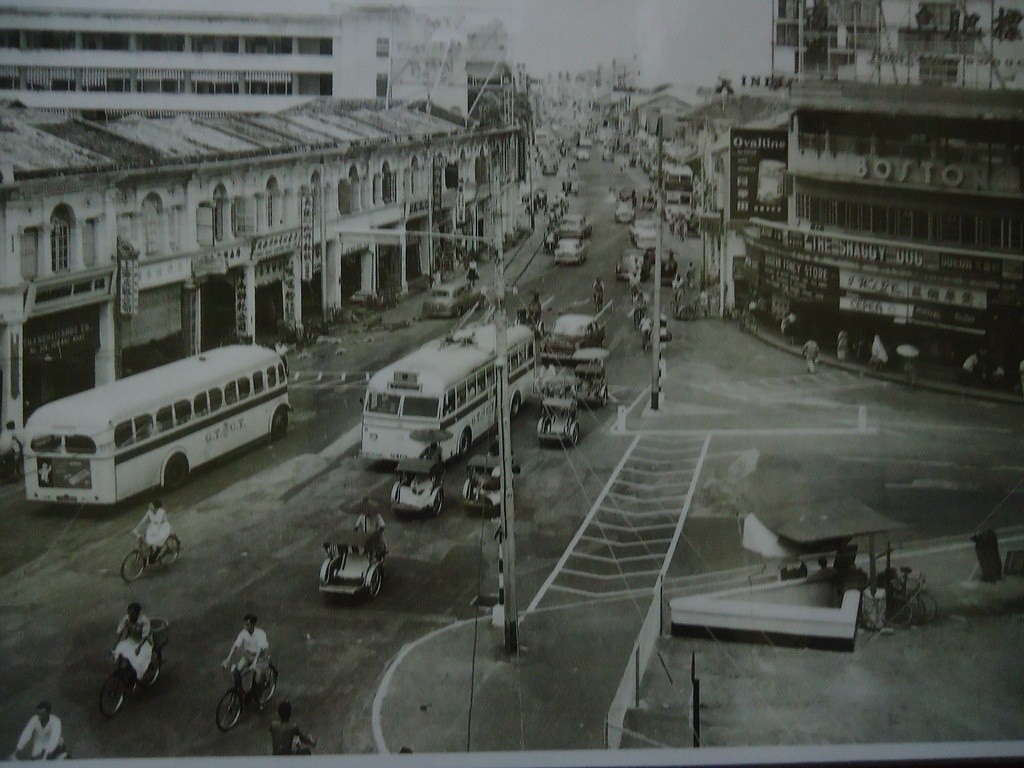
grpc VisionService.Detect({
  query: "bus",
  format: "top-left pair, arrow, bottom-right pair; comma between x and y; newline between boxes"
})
18,340 -> 295,507
356,222 -> 561,473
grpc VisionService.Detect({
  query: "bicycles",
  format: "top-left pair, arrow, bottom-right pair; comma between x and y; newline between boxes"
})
6,746 -> 72,763
593,287 -> 603,312
640,329 -> 651,353
632,303 -> 647,331
291,730 -> 317,755
96,615 -> 171,718
120,530 -> 183,584
879,566 -> 939,627
215,652 -> 280,732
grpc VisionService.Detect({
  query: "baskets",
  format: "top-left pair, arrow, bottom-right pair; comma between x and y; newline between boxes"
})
149,618 -> 169,647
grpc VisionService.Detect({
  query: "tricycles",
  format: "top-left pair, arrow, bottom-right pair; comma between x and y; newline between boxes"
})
390,426 -> 455,516
462,436 -> 521,510
570,347 -> 611,407
513,305 -> 547,341
316,496 -> 391,606
535,374 -> 582,447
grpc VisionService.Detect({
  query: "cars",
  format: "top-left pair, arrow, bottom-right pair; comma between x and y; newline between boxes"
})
541,313 -> 608,368
420,278 -> 482,320
614,248 -> 653,283
535,136 -> 660,268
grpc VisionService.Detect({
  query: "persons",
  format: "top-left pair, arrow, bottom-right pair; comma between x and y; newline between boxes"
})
533,138 -> 578,253
631,151 -> 713,240
133,498 -> 170,564
0,420 -> 23,474
9,701 -> 68,760
399,746 -> 413,754
639,312 -> 652,335
354,513 -> 387,560
628,251 -> 709,317
572,348 -> 611,360
269,700 -> 316,755
559,383 -> 576,400
529,294 -> 542,321
488,435 -> 500,454
538,357 -> 556,398
113,603 -> 154,693
466,258 -> 479,287
222,614 -> 270,710
780,312 -> 1024,395
419,441 -> 442,461
476,468 -> 489,486
592,277 -> 604,305
432,268 -> 442,290
589,354 -> 604,368
808,556 -> 840,583
274,341 -> 288,373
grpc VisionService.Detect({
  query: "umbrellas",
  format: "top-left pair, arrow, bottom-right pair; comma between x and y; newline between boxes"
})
543,374 -> 580,397
339,496 -> 389,533
409,429 -> 454,447
896,344 -> 919,368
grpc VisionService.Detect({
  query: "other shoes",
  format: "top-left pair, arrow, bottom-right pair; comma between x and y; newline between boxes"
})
150,555 -> 156,564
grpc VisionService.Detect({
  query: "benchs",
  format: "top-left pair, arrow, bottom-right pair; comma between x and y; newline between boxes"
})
333,553 -> 370,583
547,416 -> 570,434
